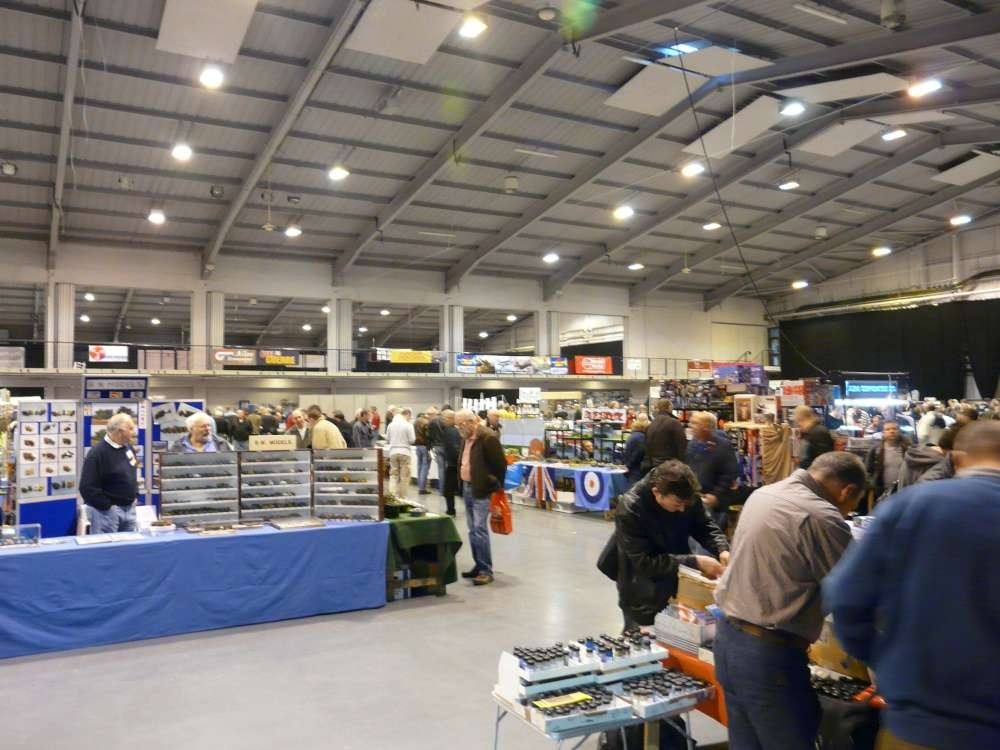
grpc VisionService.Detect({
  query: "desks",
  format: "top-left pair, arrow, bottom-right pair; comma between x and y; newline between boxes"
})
0,507 -> 463,657
632,621 -> 885,750
505,460 -> 628,518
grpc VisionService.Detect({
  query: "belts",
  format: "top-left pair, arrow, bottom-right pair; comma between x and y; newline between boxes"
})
727,616 -> 809,650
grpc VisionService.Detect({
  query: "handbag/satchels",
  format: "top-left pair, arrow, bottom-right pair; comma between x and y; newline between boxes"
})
490,489 -> 511,535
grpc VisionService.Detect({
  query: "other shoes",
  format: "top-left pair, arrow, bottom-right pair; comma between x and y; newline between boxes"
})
473,575 -> 491,585
462,569 -> 477,578
419,490 -> 431,494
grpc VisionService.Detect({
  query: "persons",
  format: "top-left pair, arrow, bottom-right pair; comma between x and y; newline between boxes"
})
616,461 -> 730,630
573,400 -> 652,425
385,405 -> 461,516
171,413 -> 230,515
625,399 -> 737,555
79,414 -> 139,534
455,409 -> 507,584
213,404 -> 380,504
479,410 -> 502,438
706,452 -> 869,750
824,419 -> 1000,750
793,400 -> 1000,515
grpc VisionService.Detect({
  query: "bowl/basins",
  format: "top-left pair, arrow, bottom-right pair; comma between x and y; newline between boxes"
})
754,414 -> 774,424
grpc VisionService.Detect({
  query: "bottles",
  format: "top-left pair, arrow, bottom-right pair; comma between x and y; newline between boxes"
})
6,512 -> 15,525
462,392 -> 540,419
512,603 -> 716,717
741,402 -> 748,421
